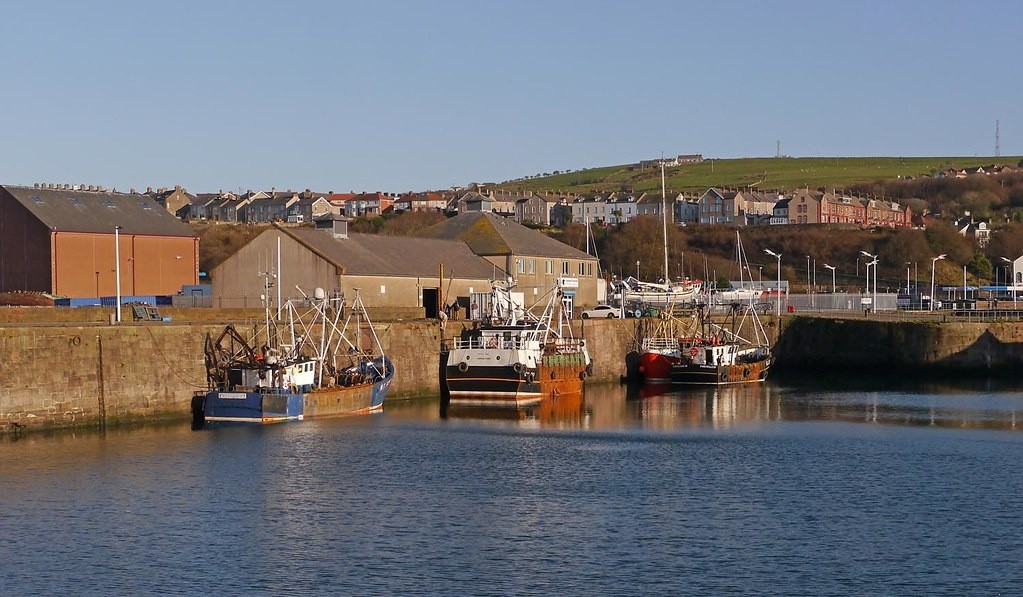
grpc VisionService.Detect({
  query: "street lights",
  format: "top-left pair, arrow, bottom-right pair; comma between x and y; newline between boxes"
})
765,243 -> 948,315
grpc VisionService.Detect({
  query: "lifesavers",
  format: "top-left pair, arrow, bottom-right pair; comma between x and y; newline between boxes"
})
712,336 -> 719,347
690,347 -> 698,356
489,338 -> 497,347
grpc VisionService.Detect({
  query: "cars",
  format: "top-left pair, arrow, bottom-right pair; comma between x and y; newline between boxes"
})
897,293 -> 1023,308
581,304 -> 622,320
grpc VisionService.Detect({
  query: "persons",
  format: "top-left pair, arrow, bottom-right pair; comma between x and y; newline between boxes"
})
471,300 -> 478,320
443,301 -> 451,320
994,297 -> 998,308
450,299 -> 460,321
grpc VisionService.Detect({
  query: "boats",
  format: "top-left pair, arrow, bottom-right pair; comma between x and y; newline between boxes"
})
632,293 -> 775,389
195,246 -> 396,429
445,274 -> 595,408
